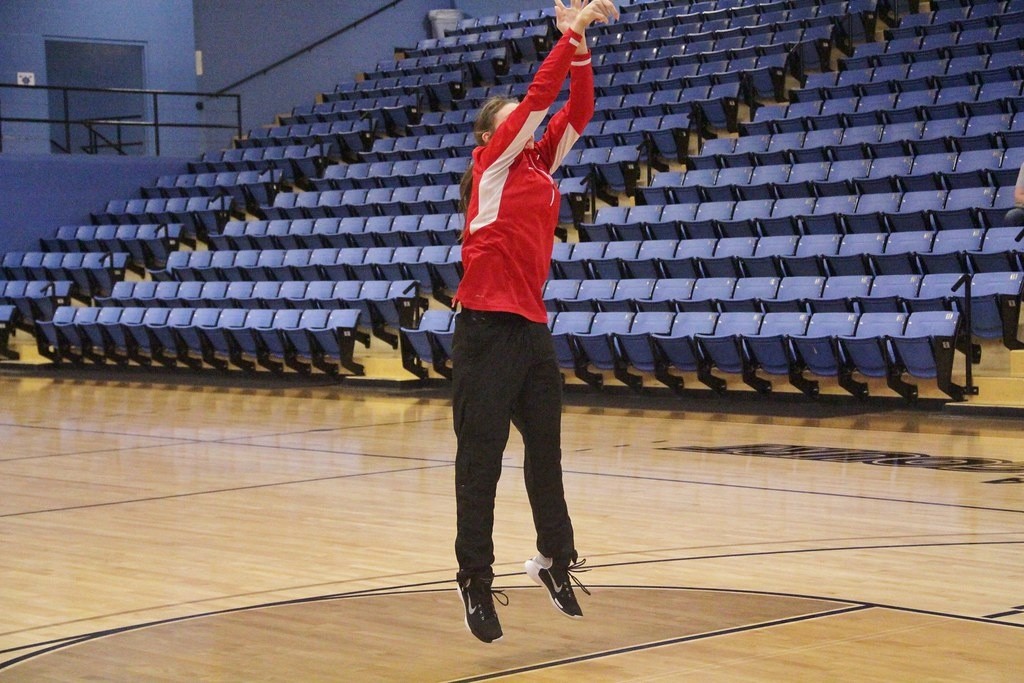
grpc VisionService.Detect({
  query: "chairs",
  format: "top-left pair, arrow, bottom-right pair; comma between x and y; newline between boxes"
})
0,0 -> 1024,400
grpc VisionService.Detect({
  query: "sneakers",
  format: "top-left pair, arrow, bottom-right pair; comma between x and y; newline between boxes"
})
525,552 -> 591,620
456,578 -> 509,644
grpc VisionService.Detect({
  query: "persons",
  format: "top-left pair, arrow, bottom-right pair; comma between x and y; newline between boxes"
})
451,0 -> 620,643
1004,163 -> 1024,226
